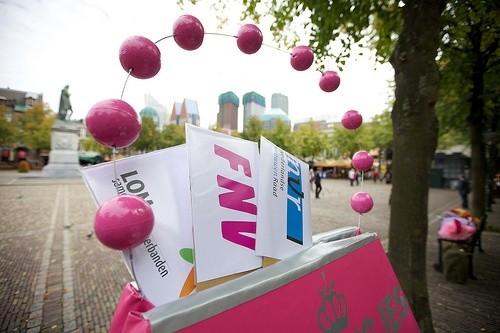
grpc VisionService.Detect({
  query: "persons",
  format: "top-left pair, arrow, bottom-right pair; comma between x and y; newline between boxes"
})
58,84 -> 73,120
308,166 -> 379,197
457,174 -> 471,208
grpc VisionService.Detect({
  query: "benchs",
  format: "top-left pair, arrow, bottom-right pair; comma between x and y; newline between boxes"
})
433,211 -> 487,281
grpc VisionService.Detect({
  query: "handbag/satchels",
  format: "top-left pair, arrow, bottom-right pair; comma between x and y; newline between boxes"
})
442,244 -> 470,282
